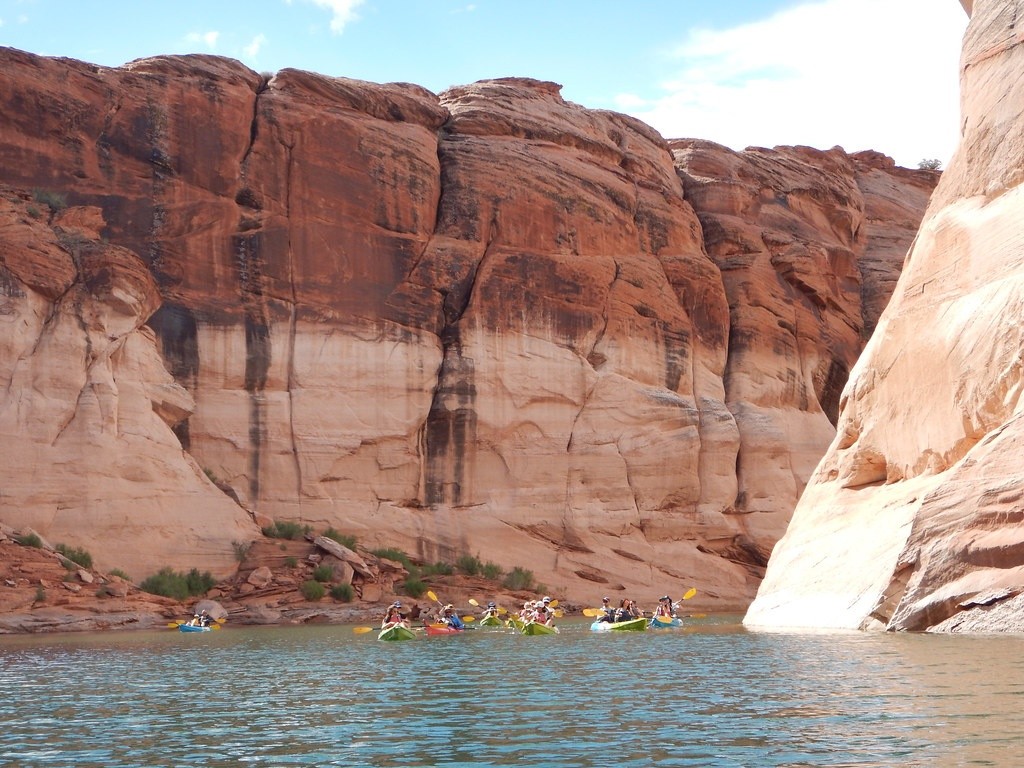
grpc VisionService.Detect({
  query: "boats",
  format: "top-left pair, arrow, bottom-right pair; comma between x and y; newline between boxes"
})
507,619 -> 525,630
523,622 -> 556,635
178,623 -> 210,633
650,614 -> 684,627
479,616 -> 503,626
378,623 -> 417,641
592,617 -> 648,631
422,619 -> 464,634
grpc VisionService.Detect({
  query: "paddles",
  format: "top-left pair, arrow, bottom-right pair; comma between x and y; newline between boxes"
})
175,618 -> 226,624
550,599 -> 559,608
167,622 -> 221,630
677,613 -> 707,619
351,623 -> 448,634
461,616 -> 475,622
497,607 -> 507,615
582,607 -> 606,618
646,588 -> 696,627
468,598 -> 486,610
554,610 -> 563,618
427,590 -> 444,607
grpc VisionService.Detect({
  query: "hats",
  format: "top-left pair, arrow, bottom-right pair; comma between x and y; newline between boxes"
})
444,606 -> 450,611
659,597 -> 662,602
448,604 -> 454,608
394,601 -> 402,608
488,602 -> 496,606
542,597 -> 550,602
603,597 -> 611,603
524,602 -> 531,611
662,595 -> 670,599
530,600 -> 537,605
533,601 -> 544,608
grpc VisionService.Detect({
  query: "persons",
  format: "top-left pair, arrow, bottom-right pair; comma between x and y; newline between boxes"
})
597,596 -> 644,622
185,609 -> 215,628
482,596 -> 553,626
434,604 -> 465,630
380,601 -> 411,629
655,595 -> 675,618
